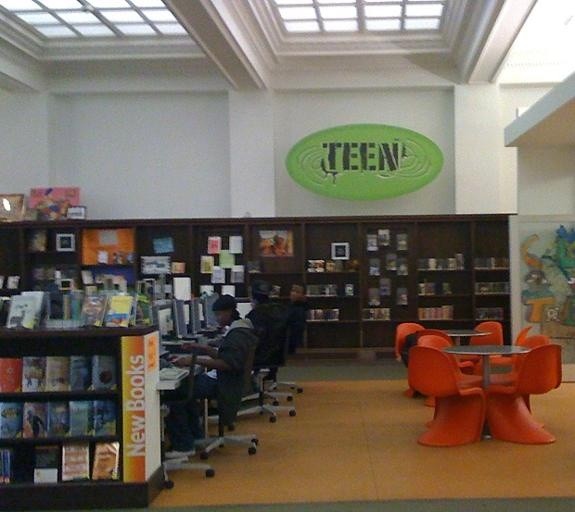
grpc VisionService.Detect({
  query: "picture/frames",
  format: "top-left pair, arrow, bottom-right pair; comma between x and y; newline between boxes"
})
330,242 -> 349,261
66,206 -> 86,220
56,233 -> 74,252
0,193 -> 25,221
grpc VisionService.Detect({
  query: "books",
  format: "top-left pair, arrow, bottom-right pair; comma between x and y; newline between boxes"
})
0,270 -> 153,484
418,254 -> 510,321
307,260 -> 355,321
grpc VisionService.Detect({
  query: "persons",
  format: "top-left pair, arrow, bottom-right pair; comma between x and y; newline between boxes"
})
245,280 -> 288,368
261,280 -> 311,382
164,294 -> 259,459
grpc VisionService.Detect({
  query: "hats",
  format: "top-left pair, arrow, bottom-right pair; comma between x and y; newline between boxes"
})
212,295 -> 237,311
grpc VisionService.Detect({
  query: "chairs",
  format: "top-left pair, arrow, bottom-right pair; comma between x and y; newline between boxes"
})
395,322 -> 563,447
161,304 -> 303,488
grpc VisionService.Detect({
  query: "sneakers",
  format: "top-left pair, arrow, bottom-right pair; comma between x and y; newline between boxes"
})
162,446 -> 197,459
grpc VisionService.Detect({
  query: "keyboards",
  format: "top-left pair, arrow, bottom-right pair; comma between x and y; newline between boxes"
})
159,365 -> 188,381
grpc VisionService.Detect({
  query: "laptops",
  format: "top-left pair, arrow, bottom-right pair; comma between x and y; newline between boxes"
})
189,297 -> 216,336
173,299 -> 203,340
205,297 -> 219,326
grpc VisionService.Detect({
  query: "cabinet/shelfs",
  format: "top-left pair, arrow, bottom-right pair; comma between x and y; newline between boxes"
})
0,214 -> 511,348
0,328 -> 164,511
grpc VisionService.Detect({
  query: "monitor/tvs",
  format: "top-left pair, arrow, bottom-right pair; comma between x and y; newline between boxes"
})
151,304 -> 161,331
237,300 -> 253,316
